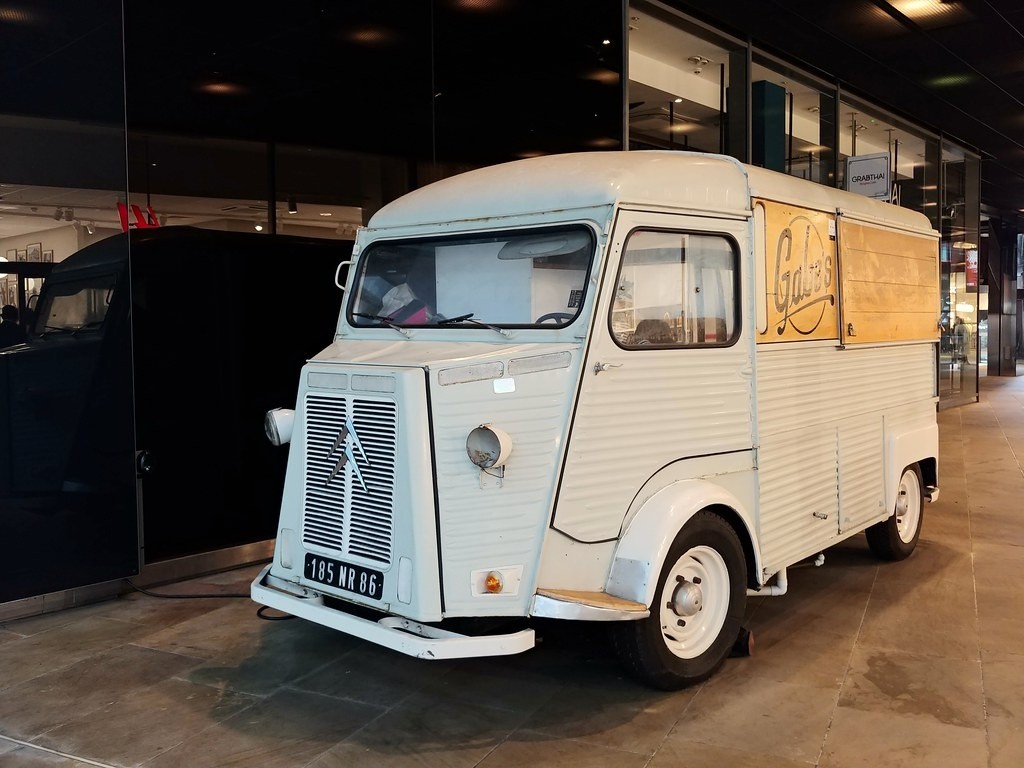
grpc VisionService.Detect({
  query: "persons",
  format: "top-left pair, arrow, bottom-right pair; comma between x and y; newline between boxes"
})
949,316 -> 969,364
0,304 -> 21,350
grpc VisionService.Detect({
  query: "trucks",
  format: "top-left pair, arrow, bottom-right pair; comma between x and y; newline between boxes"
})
249,149 -> 941,693
1,225 -> 357,560
